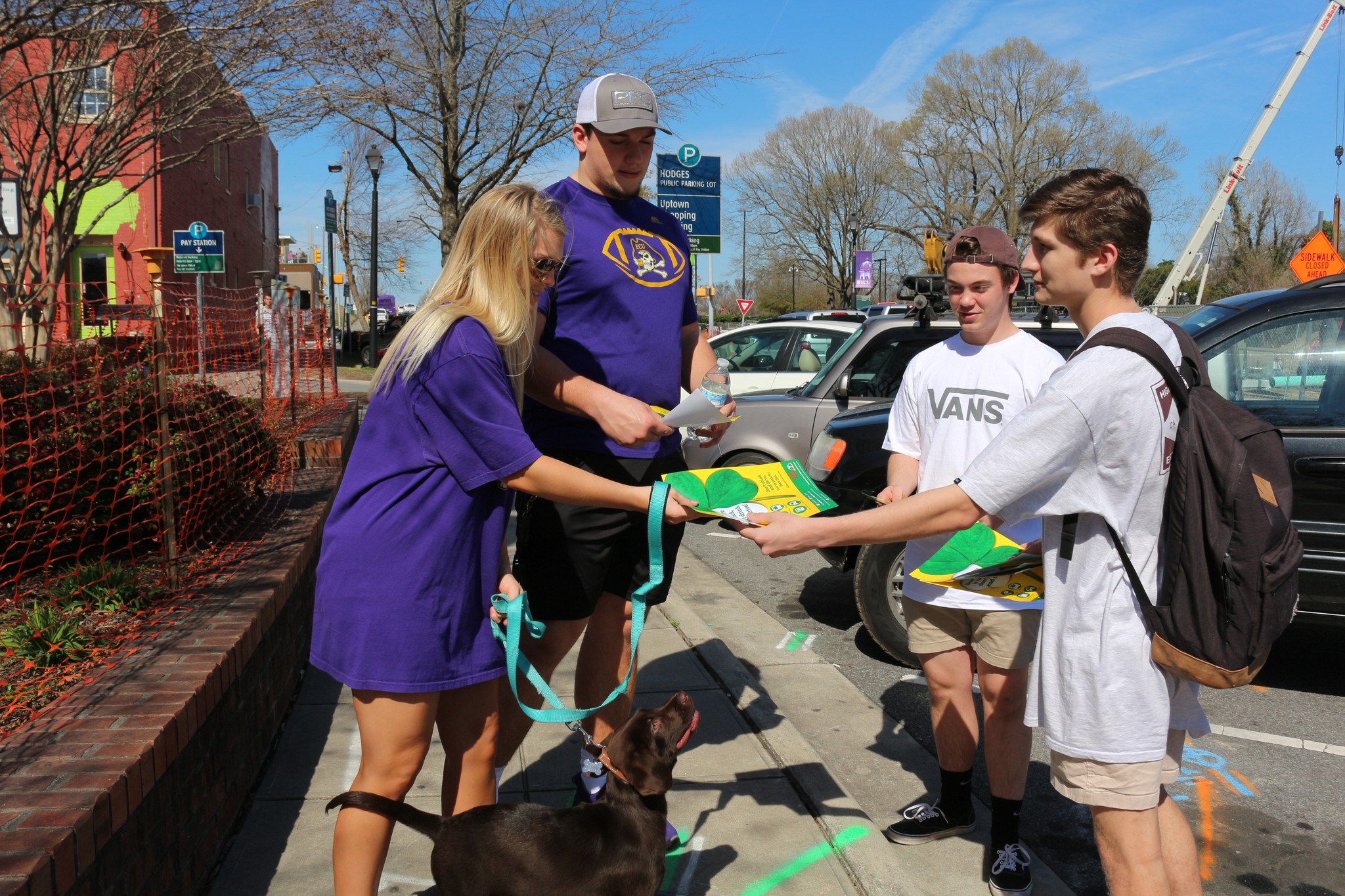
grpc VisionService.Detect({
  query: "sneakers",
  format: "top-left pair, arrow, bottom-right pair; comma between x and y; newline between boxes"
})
987,835 -> 1033,896
886,797 -> 976,845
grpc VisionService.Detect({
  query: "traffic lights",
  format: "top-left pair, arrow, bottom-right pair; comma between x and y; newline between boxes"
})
324,277 -> 327,284
313,249 -> 321,264
404,274 -> 406,280
420,281 -> 422,286
397,255 -> 405,272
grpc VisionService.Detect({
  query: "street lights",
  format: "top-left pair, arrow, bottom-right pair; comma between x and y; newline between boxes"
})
847,212 -> 861,310
737,208 -> 752,299
873,258 -> 887,303
880,249 -> 892,303
364,144 -> 384,366
788,264 -> 799,313
328,148 -> 352,355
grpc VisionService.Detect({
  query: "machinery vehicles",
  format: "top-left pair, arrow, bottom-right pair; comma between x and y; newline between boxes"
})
1142,0 -> 1345,306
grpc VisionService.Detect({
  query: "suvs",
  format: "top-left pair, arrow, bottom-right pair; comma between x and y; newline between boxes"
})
677,266 -> 1345,669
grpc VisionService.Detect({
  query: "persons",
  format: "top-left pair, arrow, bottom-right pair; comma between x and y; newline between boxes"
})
489,73 -> 738,851
296,248 -> 306,263
874,229 -> 1069,896
730,168 -> 1209,896
288,251 -> 295,264
254,295 -> 272,349
309,183 -> 713,896
705,321 -> 709,332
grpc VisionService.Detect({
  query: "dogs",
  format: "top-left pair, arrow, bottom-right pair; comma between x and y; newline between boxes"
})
325,690 -> 699,896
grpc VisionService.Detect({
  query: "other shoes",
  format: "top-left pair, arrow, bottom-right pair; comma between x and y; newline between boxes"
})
664,820 -> 679,846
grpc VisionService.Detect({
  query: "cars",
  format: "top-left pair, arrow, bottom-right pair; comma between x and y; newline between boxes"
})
368,308 -> 393,328
396,306 -> 404,316
356,312 -> 416,366
402,303 -> 418,314
94,303 -> 156,338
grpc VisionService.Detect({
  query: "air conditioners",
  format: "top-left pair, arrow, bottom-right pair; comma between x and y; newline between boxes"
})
246,193 -> 261,210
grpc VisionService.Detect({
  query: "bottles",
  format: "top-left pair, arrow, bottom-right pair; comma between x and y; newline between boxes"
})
687,358 -> 730,442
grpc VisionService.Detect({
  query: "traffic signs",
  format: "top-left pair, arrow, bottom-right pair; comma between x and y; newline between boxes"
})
173,221 -> 225,273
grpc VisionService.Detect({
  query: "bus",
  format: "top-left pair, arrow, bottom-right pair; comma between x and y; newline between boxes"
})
377,295 -> 396,317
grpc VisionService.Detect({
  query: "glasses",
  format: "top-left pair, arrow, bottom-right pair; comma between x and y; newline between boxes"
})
528,255 -> 562,279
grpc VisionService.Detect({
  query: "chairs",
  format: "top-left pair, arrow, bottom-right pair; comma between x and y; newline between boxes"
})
797,347 -> 822,372
825,346 -> 841,363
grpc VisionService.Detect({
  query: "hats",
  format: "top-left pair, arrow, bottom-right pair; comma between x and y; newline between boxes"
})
575,73 -> 672,136
943,225 -> 1026,291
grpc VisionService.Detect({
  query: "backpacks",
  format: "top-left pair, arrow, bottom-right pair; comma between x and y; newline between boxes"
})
1067,316 -> 1303,688
